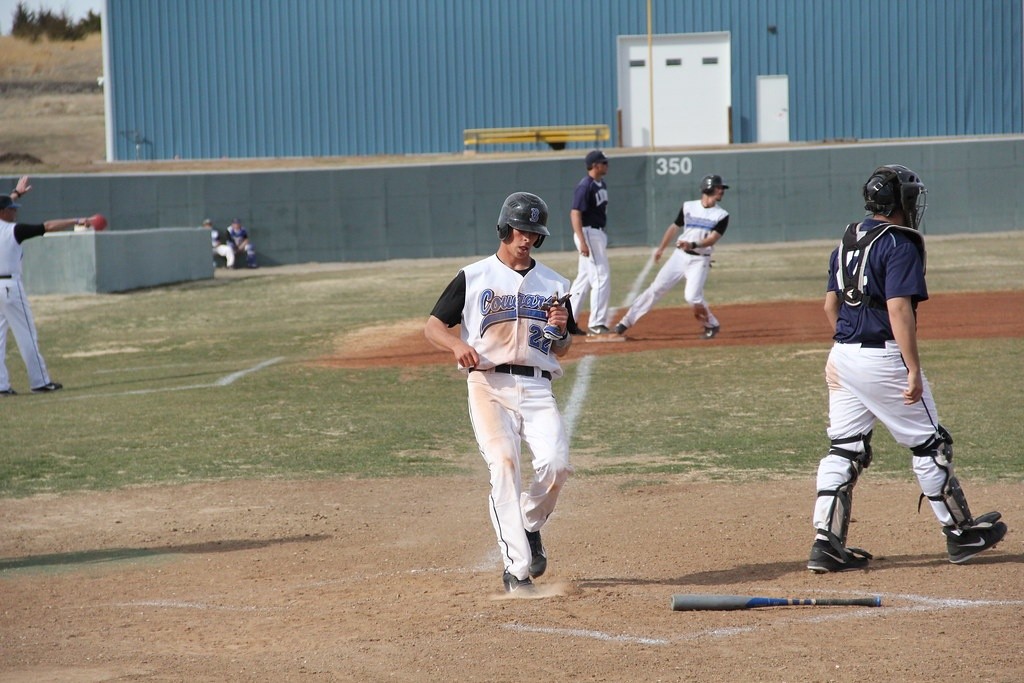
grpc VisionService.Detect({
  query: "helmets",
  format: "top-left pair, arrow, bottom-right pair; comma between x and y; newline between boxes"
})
203,219 -> 214,226
496,192 -> 548,248
233,218 -> 240,225
865,165 -> 919,211
702,175 -> 729,193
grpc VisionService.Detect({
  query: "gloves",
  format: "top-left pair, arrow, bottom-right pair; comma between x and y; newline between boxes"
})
543,291 -> 572,340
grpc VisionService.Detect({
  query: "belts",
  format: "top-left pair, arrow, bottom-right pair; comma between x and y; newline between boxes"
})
468,362 -> 552,381
590,226 -> 604,230
861,341 -> 885,348
0,275 -> 12,279
685,250 -> 712,256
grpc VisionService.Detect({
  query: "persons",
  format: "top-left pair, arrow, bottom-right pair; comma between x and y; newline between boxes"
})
424,192 -> 571,598
202,219 -> 235,269
0,175 -> 107,395
568,149 -> 616,337
806,164 -> 1008,573
226,217 -> 258,269
611,174 -> 730,339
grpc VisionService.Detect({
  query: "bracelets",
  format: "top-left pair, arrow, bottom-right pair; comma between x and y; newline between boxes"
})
692,242 -> 697,249
77,218 -> 79,224
12,189 -> 20,197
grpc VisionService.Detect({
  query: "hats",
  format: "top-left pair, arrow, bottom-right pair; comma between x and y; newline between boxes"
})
586,151 -> 611,166
1,194 -> 22,211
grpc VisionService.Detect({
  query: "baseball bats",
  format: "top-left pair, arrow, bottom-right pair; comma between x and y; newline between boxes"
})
669,593 -> 882,612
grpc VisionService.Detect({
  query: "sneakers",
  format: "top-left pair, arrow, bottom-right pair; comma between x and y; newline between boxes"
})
807,546 -> 869,573
700,326 -> 719,340
524,530 -> 547,578
1,388 -> 16,398
31,383 -> 62,394
614,323 -> 627,335
944,522 -> 1007,564
588,326 -> 609,335
503,575 -> 543,600
